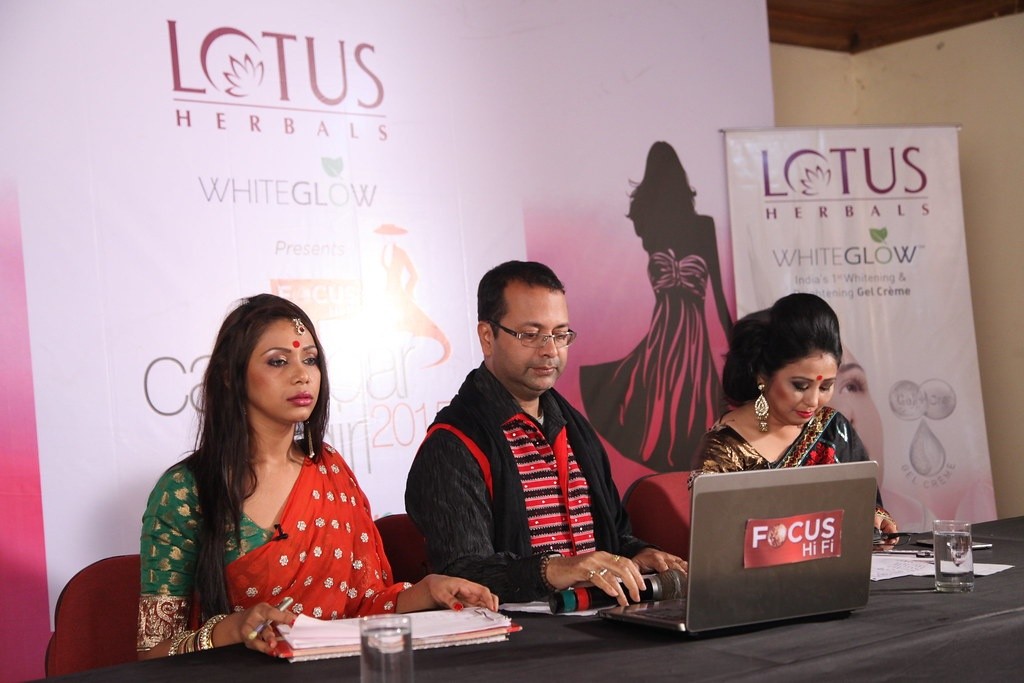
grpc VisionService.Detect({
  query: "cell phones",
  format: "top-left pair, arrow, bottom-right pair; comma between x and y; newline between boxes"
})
916,540 -> 992,550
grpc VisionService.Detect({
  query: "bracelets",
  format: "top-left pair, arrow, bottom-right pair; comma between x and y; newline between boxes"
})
169,614 -> 228,657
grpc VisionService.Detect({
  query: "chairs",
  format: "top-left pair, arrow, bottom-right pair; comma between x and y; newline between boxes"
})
46,554 -> 142,678
621,470 -> 692,563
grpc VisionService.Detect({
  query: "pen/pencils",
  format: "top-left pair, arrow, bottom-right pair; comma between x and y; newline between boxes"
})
916,552 -> 934,558
248,597 -> 293,639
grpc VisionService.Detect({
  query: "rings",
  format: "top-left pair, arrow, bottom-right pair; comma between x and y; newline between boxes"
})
611,555 -> 621,561
586,570 -> 595,581
597,568 -> 608,576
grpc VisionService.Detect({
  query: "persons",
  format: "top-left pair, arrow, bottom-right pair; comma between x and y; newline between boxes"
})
688,292 -> 900,550
406,260 -> 688,607
137,293 -> 499,659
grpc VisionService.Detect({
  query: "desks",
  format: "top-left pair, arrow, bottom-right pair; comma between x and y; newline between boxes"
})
14,516 -> 1024,683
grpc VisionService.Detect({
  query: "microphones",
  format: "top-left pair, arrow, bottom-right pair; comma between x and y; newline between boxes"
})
549,568 -> 688,614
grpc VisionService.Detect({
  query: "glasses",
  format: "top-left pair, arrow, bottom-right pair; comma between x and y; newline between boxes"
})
481,318 -> 577,350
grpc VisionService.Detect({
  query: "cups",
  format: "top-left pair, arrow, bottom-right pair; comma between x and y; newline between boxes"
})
359,613 -> 415,683
933,520 -> 975,594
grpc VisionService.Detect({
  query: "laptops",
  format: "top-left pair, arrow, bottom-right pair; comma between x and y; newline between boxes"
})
598,460 -> 879,639
